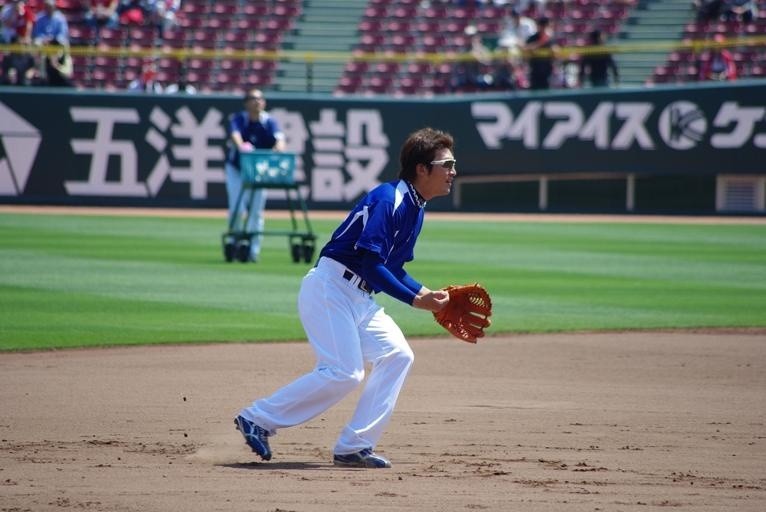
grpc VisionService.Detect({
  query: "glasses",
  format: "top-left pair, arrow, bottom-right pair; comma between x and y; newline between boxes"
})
428,158 -> 457,169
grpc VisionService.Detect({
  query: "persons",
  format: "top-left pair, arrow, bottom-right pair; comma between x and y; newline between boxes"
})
446,0 -> 620,92
0,0 -> 182,87
691,0 -> 726,29
222,88 -> 283,260
722,0 -> 758,24
698,34 -> 737,81
230,126 -> 458,470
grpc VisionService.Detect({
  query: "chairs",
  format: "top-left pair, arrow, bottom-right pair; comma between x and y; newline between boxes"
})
66,0 -> 765,93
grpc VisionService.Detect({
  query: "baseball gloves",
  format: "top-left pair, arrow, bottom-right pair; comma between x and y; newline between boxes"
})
431,283 -> 491,344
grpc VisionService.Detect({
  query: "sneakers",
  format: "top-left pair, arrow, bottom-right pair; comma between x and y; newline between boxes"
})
233,412 -> 272,461
333,445 -> 392,468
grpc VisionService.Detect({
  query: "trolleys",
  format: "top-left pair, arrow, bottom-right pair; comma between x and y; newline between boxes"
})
223,148 -> 315,262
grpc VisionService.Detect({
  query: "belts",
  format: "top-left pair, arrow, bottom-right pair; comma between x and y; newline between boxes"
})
312,256 -> 374,296
